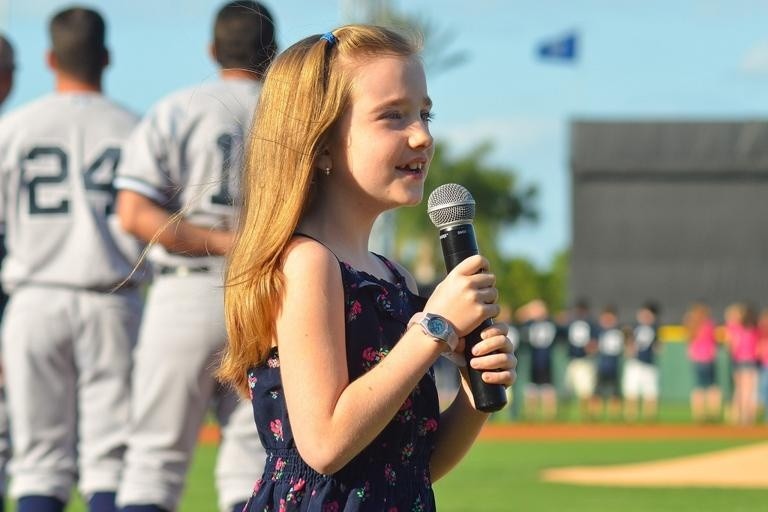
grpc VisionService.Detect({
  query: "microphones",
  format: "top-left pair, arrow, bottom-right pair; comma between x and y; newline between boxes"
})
425,182 -> 508,413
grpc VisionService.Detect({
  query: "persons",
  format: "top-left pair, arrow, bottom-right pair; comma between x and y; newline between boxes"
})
1,7 -> 140,512
214,24 -> 517,512
113,0 -> 276,512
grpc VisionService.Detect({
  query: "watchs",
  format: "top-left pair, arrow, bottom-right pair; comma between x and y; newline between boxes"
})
406,311 -> 458,352
